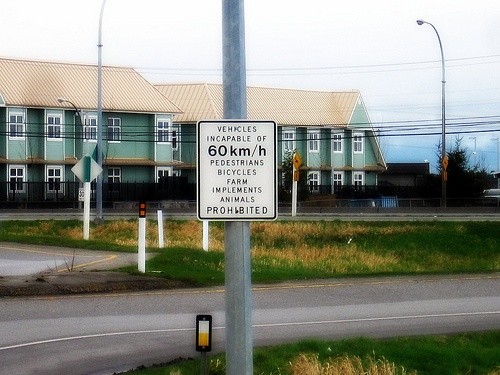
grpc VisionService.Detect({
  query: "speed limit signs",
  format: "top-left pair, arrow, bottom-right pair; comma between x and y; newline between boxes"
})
194,119 -> 278,222
78,188 -> 84,202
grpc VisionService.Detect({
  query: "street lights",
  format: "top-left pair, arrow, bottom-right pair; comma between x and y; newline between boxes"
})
417,19 -> 448,207
57,97 -> 82,210
